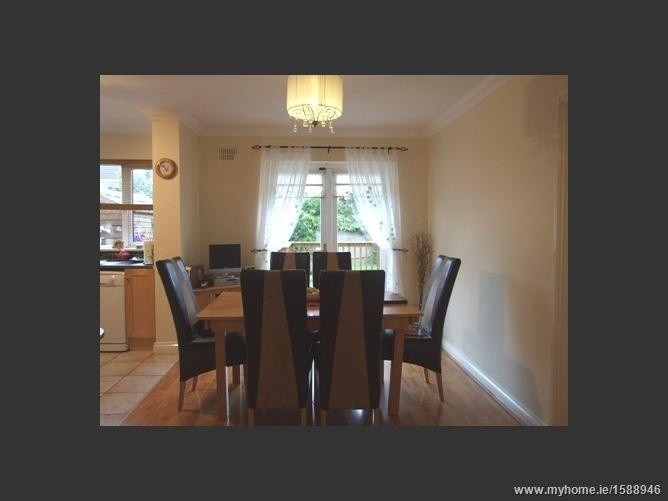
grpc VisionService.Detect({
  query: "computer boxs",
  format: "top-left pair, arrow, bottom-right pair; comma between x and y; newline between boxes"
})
210,276 -> 240,286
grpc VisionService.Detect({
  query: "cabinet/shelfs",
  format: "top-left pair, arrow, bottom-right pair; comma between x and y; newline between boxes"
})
123,269 -> 156,351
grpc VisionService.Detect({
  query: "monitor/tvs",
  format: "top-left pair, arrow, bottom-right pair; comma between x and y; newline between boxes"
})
205,239 -> 244,278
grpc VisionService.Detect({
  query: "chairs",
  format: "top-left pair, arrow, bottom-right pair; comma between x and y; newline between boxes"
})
155,249 -> 461,427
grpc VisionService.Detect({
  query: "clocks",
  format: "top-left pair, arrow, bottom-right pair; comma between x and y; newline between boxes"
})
155,158 -> 177,179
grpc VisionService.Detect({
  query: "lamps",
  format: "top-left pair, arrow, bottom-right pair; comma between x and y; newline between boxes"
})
284,76 -> 343,136
